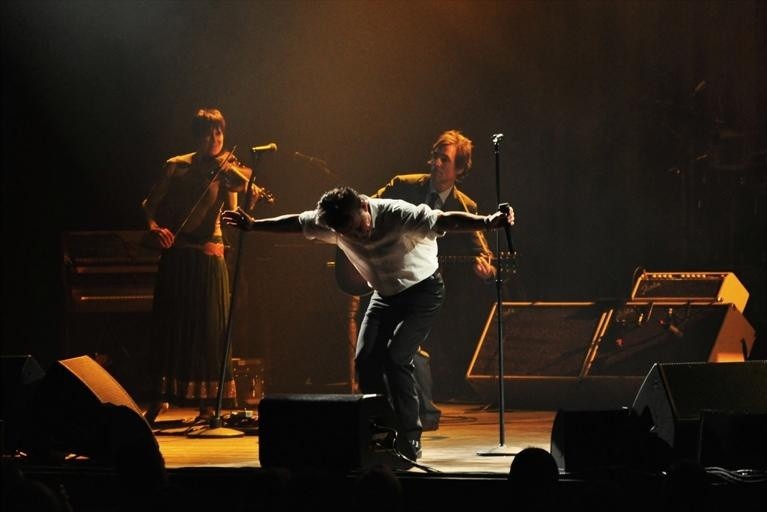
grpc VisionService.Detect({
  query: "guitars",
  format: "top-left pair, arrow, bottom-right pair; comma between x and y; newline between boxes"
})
334,244 -> 520,297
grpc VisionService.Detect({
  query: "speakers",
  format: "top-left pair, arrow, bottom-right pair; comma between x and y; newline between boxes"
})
464,298 -> 609,406
632,360 -> 767,467
256,390 -> 416,472
580,302 -> 757,398
41,353 -> 145,417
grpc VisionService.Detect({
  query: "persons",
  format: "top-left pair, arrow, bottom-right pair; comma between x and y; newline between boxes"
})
368,130 -> 495,432
220,188 -> 516,460
139,109 -> 260,430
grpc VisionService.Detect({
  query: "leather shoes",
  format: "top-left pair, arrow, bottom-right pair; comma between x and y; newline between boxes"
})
409,437 -> 421,458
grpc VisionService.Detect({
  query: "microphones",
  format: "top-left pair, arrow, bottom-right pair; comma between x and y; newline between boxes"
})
499,202 -> 516,254
250,142 -> 278,153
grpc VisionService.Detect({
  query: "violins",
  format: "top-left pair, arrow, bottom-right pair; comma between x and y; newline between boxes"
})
204,148 -> 276,205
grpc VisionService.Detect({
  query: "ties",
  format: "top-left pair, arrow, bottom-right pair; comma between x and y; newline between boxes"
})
428,192 -> 438,210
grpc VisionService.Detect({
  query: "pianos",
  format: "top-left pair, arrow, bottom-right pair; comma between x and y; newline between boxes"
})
58,219 -> 164,315
